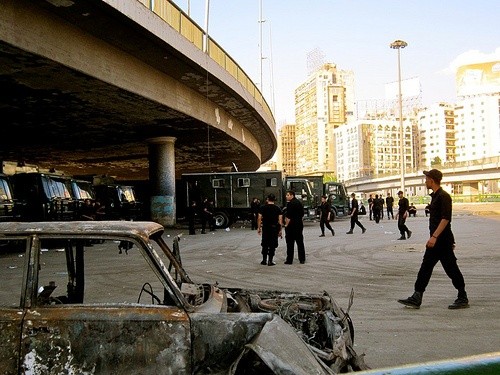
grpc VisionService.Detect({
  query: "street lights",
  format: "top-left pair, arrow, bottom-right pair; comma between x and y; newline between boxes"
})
389,38 -> 409,198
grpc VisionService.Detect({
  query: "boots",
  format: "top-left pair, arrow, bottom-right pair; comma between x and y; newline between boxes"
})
398,234 -> 405,240
449,291 -> 468,308
407,230 -> 411,239
347,229 -> 353,234
362,228 -> 366,233
398,291 -> 422,309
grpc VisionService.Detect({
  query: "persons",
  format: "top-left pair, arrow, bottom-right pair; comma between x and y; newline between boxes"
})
198,196 -> 216,234
358,204 -> 367,215
408,202 -> 417,217
186,201 -> 197,235
80,199 -> 120,247
386,193 -> 394,220
379,194 -> 384,220
372,194 -> 382,223
257,194 -> 282,267
395,169 -> 468,309
283,191 -> 305,264
346,193 -> 366,234
424,202 -> 430,217
368,195 -> 375,221
318,196 -> 335,237
395,191 -> 411,240
251,198 -> 261,231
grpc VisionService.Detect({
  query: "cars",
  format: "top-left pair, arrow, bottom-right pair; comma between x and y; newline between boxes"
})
0,221 -> 368,375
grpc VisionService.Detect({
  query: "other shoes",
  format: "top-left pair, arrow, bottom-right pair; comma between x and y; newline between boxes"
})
319,233 -> 325,237
331,230 -> 334,236
268,261 -> 276,265
261,260 -> 266,264
300,260 -> 304,264
285,261 -> 292,264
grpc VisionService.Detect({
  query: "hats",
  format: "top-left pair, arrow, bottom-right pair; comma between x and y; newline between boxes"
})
397,191 -> 403,194
423,169 -> 442,181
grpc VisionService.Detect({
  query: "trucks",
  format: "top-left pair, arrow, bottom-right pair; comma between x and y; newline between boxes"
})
285,176 -> 351,217
181,170 -> 317,229
0,170 -> 143,250
